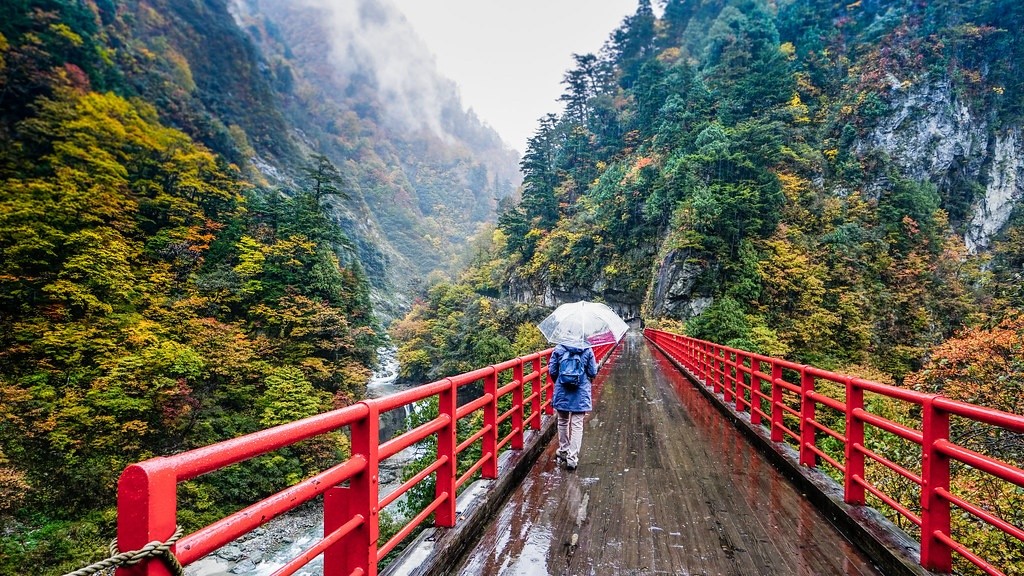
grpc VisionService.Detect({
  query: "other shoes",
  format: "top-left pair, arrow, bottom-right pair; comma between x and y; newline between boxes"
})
567,462 -> 576,470
556,448 -> 569,460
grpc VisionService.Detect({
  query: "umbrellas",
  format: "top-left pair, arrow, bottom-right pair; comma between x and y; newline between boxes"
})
537,300 -> 630,351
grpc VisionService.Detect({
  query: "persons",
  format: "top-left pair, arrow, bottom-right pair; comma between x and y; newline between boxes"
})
549,330 -> 597,470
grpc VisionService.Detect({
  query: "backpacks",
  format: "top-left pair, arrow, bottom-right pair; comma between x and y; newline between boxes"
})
559,351 -> 584,387
